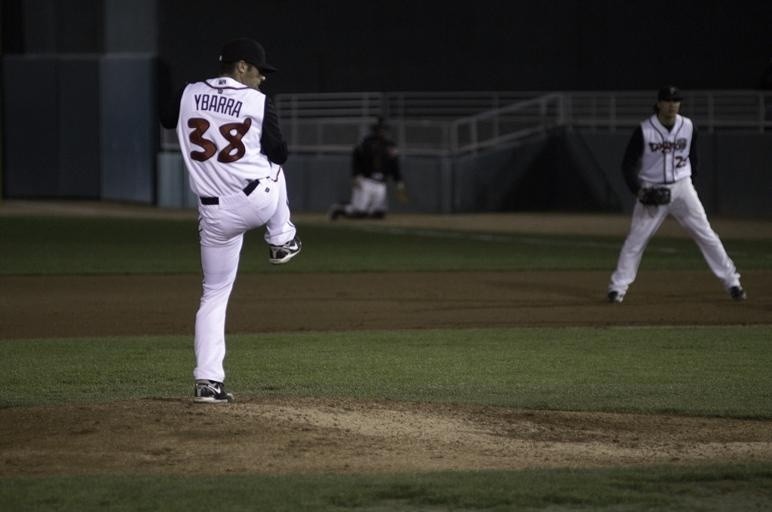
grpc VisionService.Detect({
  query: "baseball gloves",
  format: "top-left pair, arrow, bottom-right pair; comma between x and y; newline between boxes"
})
639,186 -> 670,204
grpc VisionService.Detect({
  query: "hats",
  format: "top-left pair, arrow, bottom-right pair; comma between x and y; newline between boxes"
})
222,37 -> 278,79
658,84 -> 684,101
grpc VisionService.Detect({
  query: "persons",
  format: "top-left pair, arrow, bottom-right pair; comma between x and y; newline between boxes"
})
175,37 -> 301,404
327,132 -> 406,222
606,85 -> 747,304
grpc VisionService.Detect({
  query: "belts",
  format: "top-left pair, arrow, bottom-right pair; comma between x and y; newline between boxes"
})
200,179 -> 260,205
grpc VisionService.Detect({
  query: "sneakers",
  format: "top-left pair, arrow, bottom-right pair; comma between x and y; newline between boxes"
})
729,286 -> 748,301
267,236 -> 302,265
607,290 -> 627,304
192,380 -> 234,403
